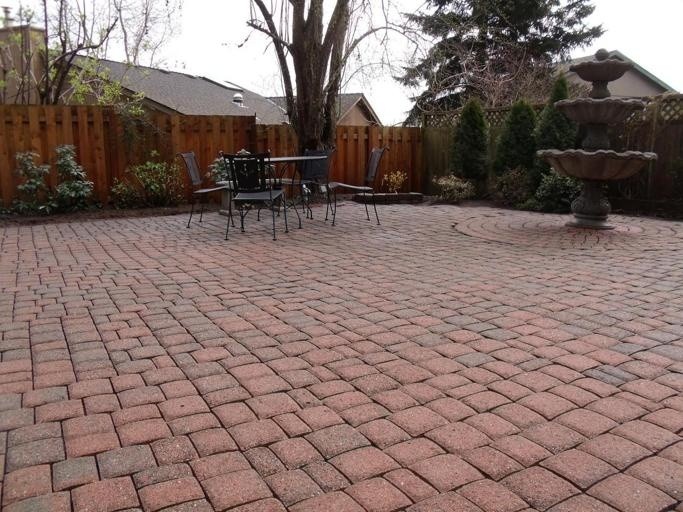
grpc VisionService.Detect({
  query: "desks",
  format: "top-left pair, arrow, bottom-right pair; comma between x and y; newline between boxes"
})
232,155 -> 328,233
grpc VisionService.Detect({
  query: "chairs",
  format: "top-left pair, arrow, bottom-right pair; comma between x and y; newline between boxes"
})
278,143 -> 336,219
325,144 -> 390,227
218,147 -> 288,241
176,150 -> 236,228
